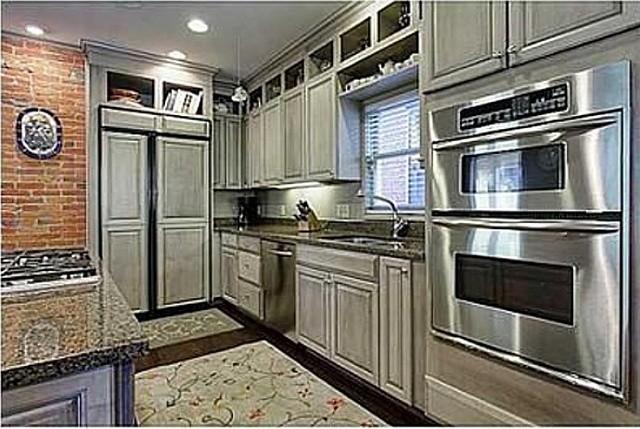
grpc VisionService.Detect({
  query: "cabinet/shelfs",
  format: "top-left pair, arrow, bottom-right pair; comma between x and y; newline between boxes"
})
420,374 -> 540,426
220,233 -> 238,308
243,99 -> 283,189
237,236 -> 261,323
282,76 -> 362,185
336,1 -> 419,100
292,245 -> 380,388
213,91 -> 240,116
378,255 -> 416,408
240,33 -> 335,120
98,66 -> 211,315
213,115 -> 243,191
418,1 -> 637,94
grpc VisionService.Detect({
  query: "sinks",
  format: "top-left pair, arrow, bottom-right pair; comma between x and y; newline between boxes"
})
318,235 -> 413,251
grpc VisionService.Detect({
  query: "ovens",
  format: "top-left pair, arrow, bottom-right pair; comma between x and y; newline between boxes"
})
426,57 -> 632,217
426,217 -> 631,408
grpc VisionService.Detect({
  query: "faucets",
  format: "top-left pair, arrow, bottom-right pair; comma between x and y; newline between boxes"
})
354,190 -> 410,237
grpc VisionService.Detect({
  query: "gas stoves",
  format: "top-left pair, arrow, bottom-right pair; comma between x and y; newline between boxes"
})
4,245 -> 96,293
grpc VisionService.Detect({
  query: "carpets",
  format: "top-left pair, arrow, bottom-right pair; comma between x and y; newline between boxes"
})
132,338 -> 392,427
139,305 -> 246,351
